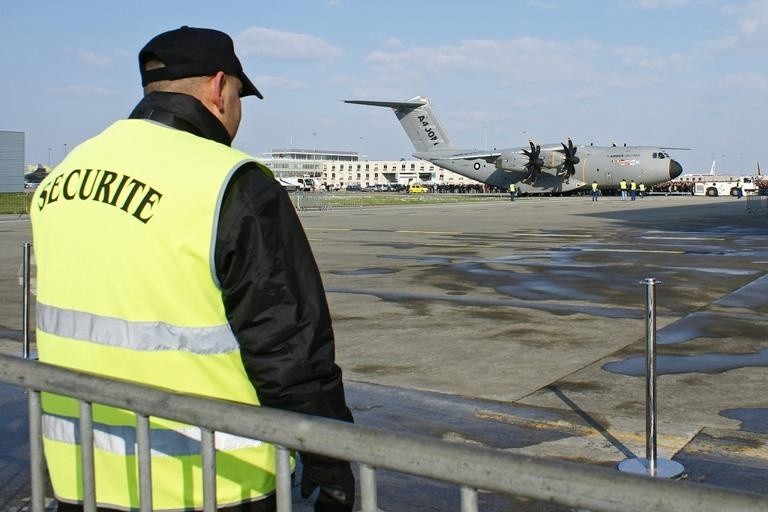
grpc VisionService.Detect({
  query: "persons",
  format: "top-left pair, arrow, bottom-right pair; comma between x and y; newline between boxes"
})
432,182 -> 503,193
590,180 -> 599,202
628,179 -> 637,201
638,181 -> 645,198
26,22 -> 359,512
618,178 -> 628,200
646,181 -> 694,196
509,182 -> 518,201
736,179 -> 742,199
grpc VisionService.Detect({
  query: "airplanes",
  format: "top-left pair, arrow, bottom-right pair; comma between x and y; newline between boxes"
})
346,93 -> 694,200
23,162 -> 49,189
677,158 -> 767,181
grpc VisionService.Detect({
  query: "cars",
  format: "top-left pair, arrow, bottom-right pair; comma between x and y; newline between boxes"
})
276,173 -> 431,195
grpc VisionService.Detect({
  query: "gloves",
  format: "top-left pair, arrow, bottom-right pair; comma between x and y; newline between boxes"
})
300,461 -> 355,512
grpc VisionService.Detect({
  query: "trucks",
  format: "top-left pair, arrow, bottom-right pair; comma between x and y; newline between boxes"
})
692,174 -> 762,198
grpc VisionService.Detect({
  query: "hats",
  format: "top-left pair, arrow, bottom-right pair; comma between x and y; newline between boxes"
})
138,25 -> 264,100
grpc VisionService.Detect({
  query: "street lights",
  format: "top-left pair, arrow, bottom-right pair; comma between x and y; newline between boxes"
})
482,124 -> 490,151
25,125 -> 67,169
